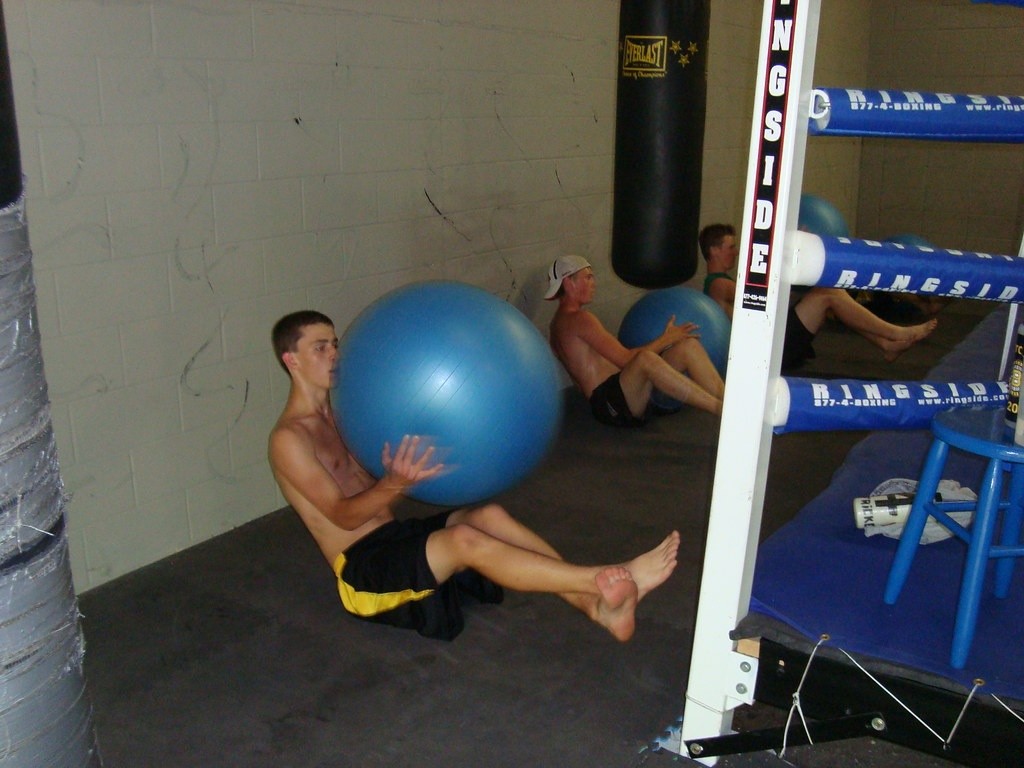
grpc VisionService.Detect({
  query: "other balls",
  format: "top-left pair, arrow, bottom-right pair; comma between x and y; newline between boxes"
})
797,194 -> 847,237
885,235 -> 931,247
330,282 -> 562,507
620,287 -> 731,385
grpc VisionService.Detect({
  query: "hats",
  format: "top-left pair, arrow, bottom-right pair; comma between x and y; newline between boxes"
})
544,254 -> 592,299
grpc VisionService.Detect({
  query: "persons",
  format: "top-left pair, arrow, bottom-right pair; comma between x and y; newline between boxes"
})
546,254 -> 724,428
269,310 -> 680,642
699,224 -> 937,364
857,290 -> 954,316
